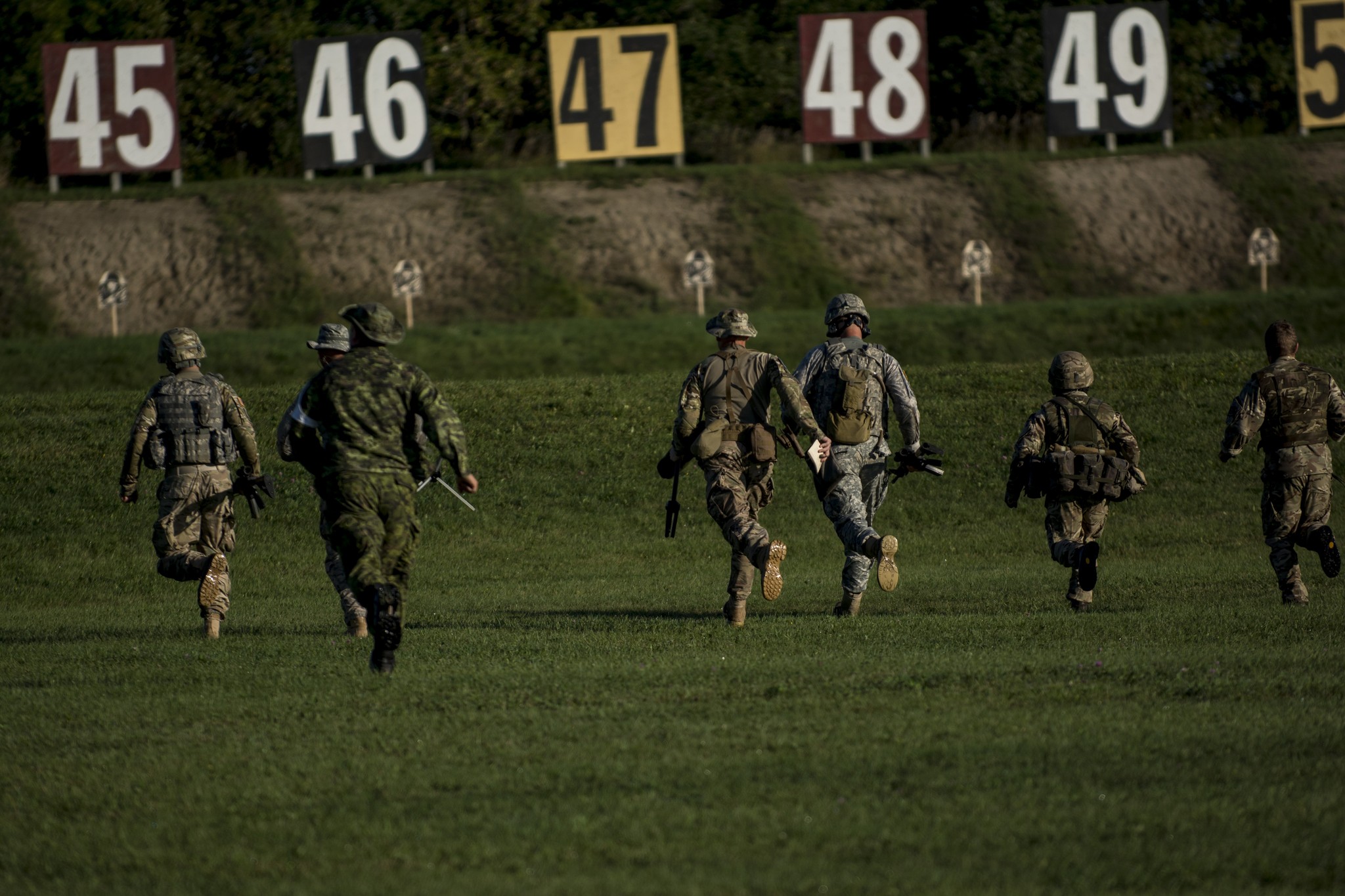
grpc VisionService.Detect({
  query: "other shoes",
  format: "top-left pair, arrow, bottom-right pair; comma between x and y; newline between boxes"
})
1074,541 -> 1099,591
1070,600 -> 1089,613
365,582 -> 402,651
368,646 -> 395,675
1313,526 -> 1341,579
342,617 -> 368,637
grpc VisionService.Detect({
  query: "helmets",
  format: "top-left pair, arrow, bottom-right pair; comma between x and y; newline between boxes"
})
157,327 -> 206,363
1048,351 -> 1095,391
825,293 -> 870,323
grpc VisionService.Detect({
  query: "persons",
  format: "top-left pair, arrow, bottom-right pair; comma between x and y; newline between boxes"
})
275,302 -> 479,679
1217,319 -> 1345,606
780,292 -> 921,619
119,327 -> 261,641
669,308 -> 831,627
1005,350 -> 1140,613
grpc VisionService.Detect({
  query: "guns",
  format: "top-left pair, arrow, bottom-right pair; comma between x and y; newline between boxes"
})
889,441 -> 945,484
233,465 -> 275,519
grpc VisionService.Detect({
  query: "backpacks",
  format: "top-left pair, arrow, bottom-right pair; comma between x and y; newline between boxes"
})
826,364 -> 871,446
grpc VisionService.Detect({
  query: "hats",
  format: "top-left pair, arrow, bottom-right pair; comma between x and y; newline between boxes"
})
706,310 -> 758,339
338,303 -> 403,344
306,325 -> 351,353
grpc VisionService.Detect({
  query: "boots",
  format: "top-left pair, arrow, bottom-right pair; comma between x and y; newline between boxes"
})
758,539 -> 787,601
872,535 -> 899,592
198,613 -> 222,639
833,590 -> 862,618
197,552 -> 226,606
722,598 -> 746,626
